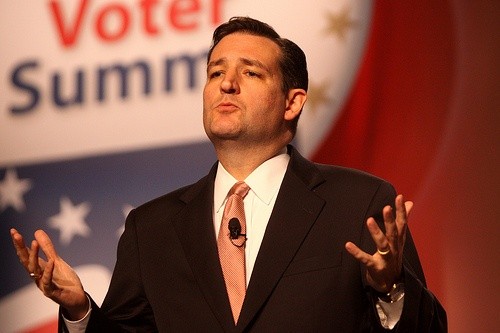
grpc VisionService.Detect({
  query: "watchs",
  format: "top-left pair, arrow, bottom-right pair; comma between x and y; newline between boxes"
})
378,283 -> 405,303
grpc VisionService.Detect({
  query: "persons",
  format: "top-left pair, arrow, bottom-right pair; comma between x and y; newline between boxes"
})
10,18 -> 447,333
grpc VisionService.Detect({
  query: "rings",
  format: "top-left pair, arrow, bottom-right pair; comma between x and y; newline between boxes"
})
28,272 -> 42,280
377,249 -> 389,255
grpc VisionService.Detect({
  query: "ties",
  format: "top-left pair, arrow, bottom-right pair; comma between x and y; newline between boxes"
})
217,181 -> 250,325
